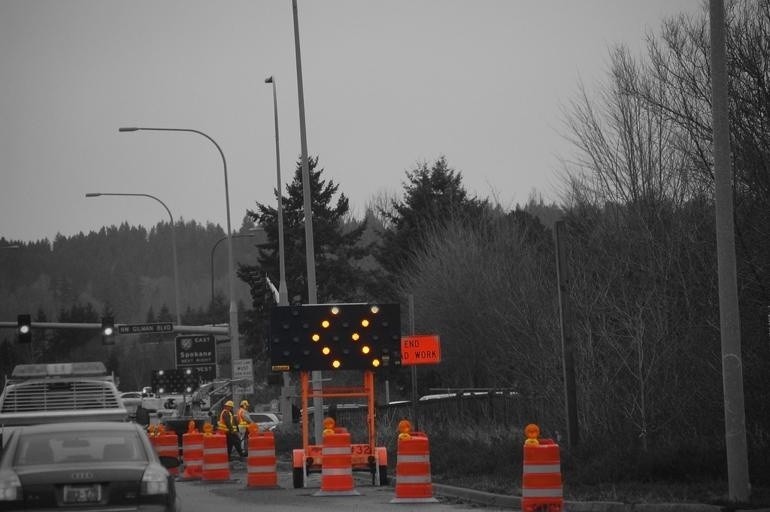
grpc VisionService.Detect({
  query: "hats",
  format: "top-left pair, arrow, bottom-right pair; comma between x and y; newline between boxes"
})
224,401 -> 234,407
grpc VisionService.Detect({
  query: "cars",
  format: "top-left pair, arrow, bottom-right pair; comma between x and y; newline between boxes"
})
0,362 -> 211,512
230,412 -> 278,434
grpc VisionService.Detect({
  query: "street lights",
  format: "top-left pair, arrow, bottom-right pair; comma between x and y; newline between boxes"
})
85,190 -> 187,345
258,71 -> 292,308
116,118 -> 245,432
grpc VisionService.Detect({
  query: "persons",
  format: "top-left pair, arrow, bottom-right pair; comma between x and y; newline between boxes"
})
220,401 -> 248,461
237,400 -> 255,456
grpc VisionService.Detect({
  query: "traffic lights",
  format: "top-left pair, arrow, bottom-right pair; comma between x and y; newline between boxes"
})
102,315 -> 114,340
15,314 -> 33,346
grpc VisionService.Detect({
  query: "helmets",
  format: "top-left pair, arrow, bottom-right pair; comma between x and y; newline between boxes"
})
240,401 -> 249,406
